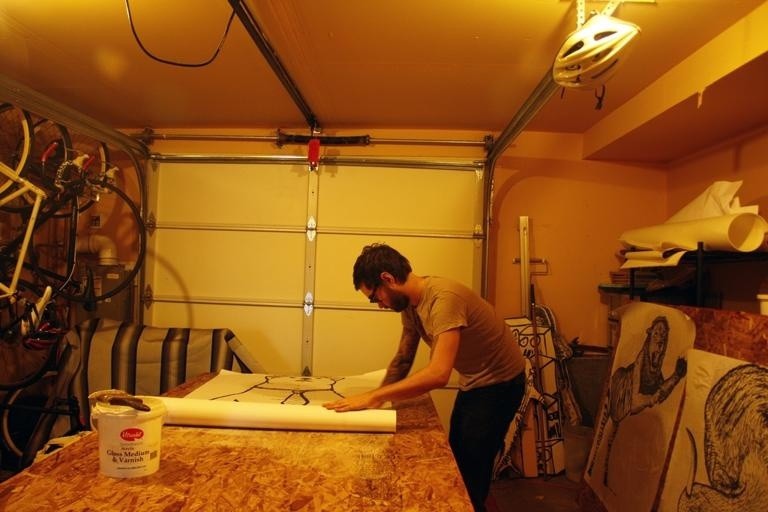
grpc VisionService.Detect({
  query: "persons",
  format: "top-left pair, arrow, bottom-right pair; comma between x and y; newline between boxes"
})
324,242 -> 526,512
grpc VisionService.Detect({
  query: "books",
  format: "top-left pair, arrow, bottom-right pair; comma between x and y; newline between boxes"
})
608,268 -> 671,288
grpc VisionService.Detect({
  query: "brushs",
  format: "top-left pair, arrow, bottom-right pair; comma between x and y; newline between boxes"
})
88,389 -> 151,411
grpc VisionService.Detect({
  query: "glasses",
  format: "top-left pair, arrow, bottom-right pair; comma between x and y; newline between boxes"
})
370,284 -> 380,303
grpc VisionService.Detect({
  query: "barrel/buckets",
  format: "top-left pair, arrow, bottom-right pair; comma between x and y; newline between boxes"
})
89,397 -> 168,478
